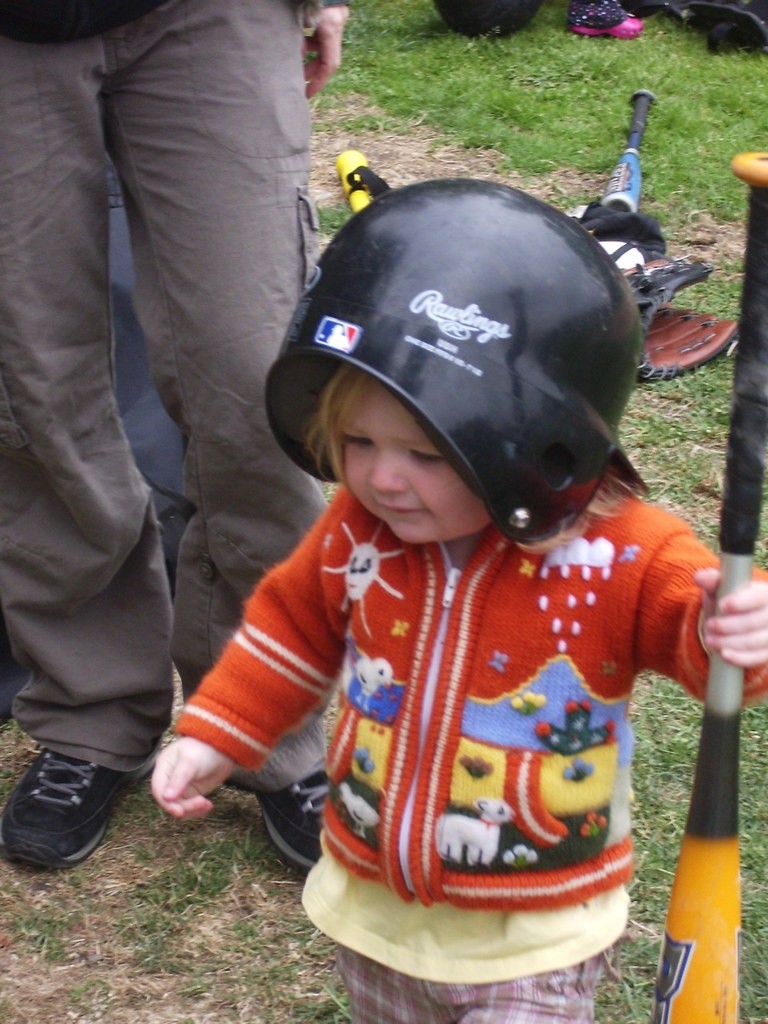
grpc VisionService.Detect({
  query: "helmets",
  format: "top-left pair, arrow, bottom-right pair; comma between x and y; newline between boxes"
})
265,178 -> 642,543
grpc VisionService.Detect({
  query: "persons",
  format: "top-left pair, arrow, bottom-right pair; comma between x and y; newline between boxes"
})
0,0 -> 348,869
152,178 -> 768,1024
568,0 -> 643,39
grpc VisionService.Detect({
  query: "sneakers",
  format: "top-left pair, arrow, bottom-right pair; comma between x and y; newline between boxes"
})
0,734 -> 160,868
223,769 -> 329,870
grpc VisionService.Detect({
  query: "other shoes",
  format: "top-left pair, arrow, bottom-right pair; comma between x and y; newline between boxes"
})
571,12 -> 643,39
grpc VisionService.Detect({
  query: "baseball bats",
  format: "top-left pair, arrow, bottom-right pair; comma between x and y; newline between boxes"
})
649,151 -> 768,1024
604,88 -> 660,215
